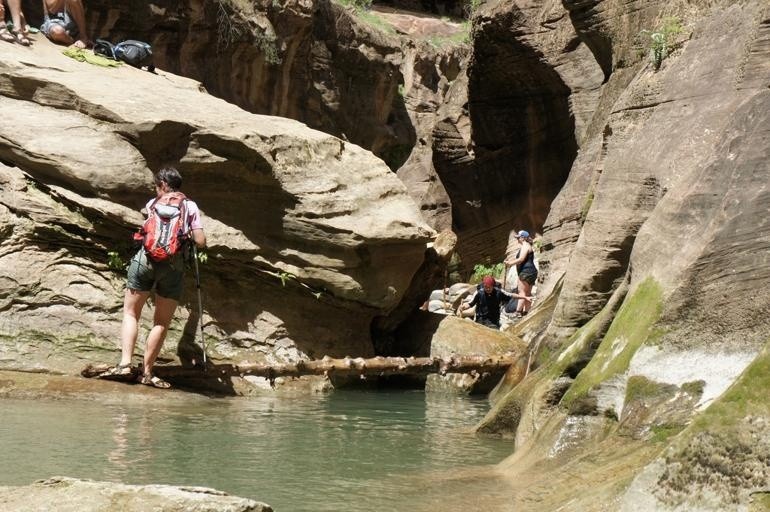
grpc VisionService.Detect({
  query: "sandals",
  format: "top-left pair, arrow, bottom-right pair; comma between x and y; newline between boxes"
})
100,364 -> 134,377
1,18 -> 30,47
138,371 -> 172,389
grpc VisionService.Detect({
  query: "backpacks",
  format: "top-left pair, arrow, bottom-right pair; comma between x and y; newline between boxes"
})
476,282 -> 501,310
140,191 -> 190,263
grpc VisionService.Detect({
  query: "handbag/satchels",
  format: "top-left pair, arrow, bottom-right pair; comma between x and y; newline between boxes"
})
93,37 -> 156,72
505,287 -> 518,313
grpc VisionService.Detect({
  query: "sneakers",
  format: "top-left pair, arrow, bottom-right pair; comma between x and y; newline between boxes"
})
514,311 -> 523,318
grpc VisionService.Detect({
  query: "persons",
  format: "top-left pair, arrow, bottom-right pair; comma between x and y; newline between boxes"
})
0,0 -> 30,46
100,166 -> 206,388
504,230 -> 538,319
24,0 -> 90,49
459,275 -> 532,330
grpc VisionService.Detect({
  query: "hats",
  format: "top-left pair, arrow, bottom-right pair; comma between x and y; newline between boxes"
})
514,230 -> 530,238
484,278 -> 495,285
